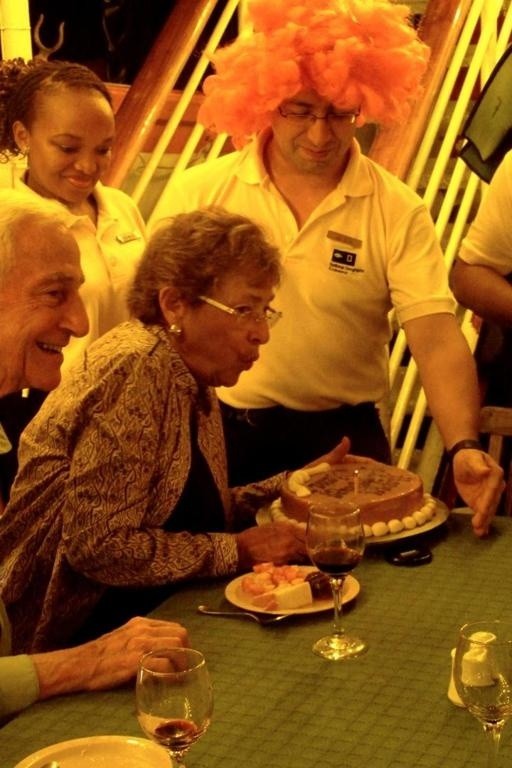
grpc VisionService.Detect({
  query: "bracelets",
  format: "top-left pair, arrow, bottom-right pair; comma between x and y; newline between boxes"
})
447,438 -> 488,459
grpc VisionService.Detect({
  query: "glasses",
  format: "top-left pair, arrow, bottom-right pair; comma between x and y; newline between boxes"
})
199,295 -> 282,329
277,105 -> 361,127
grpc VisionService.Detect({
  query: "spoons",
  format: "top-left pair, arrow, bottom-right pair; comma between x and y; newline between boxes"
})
196,603 -> 290,626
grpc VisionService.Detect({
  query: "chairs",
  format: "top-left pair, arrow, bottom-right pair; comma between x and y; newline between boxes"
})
438,406 -> 512,517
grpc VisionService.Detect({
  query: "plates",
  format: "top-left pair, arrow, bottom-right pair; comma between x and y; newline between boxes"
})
14,735 -> 173,768
225,566 -> 359,615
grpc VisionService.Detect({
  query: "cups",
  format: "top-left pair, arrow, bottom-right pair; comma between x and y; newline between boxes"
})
448,647 -> 475,708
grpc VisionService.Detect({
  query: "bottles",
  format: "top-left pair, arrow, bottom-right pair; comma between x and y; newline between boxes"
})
461,632 -> 497,687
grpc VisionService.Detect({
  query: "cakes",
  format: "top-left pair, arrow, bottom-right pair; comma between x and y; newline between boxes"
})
269,462 -> 438,539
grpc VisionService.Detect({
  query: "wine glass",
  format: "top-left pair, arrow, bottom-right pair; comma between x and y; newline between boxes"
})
135,647 -> 214,768
305,501 -> 369,660
454,619 -> 512,767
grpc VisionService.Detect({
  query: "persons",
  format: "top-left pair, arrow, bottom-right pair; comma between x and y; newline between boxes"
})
148,2 -> 506,537
1,56 -> 156,397
430,144 -> 512,517
0,204 -> 379,657
0,186 -> 191,713
